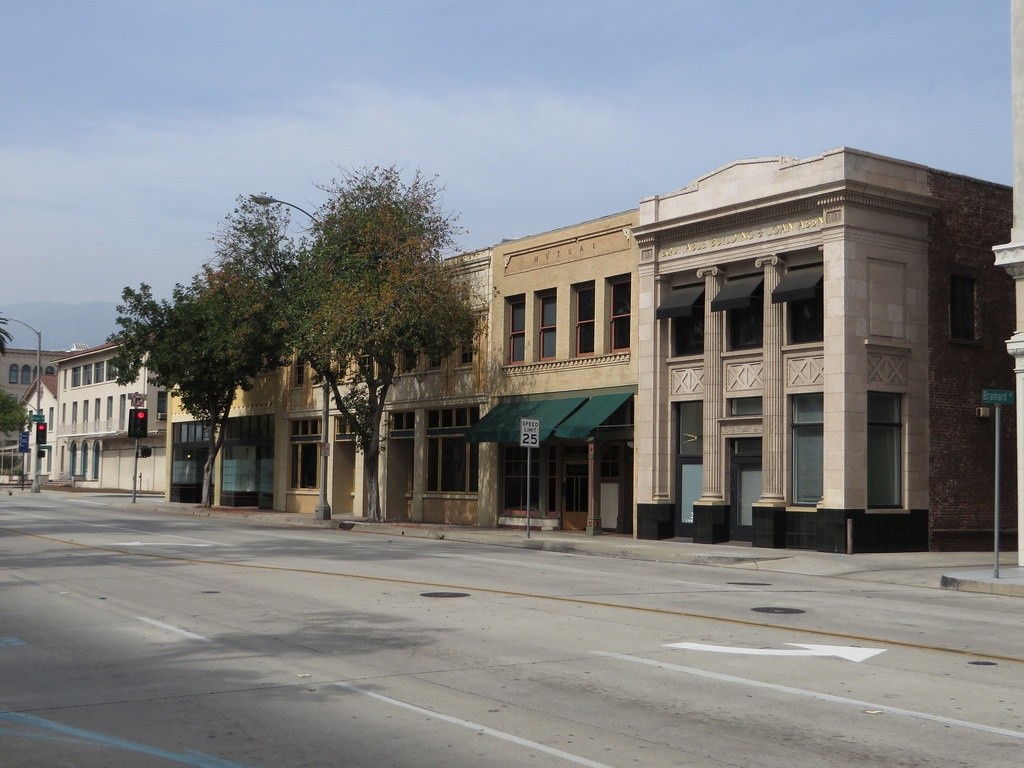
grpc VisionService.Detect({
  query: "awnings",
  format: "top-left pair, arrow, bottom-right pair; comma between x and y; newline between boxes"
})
465,393 -> 633,445
655,266 -> 823,318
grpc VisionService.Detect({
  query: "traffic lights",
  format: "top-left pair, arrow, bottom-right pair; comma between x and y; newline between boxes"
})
37,423 -> 47,444
128,409 -> 147,438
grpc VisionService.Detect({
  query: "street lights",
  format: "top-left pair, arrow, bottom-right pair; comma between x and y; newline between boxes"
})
250,194 -> 332,520
